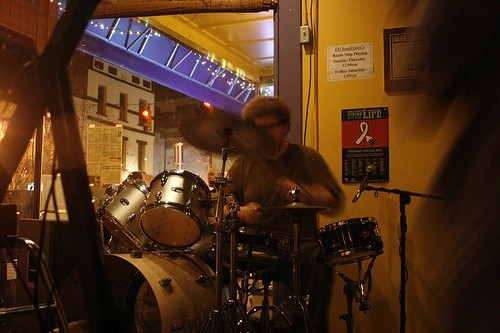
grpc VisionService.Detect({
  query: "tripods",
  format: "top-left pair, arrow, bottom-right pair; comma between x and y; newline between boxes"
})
199,130 -> 312,333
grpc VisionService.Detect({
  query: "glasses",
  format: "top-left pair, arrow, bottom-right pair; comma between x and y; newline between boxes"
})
262,122 -> 282,130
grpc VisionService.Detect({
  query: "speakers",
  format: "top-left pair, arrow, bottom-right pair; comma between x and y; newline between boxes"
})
13,217 -> 89,333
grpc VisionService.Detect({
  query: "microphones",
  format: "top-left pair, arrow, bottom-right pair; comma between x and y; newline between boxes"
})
354,165 -> 377,201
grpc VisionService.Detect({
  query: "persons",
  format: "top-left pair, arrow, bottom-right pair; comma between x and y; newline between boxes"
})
222,96 -> 344,332
192,167 -> 222,258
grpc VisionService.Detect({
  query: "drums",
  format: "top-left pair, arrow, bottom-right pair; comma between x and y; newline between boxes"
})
58,250 -> 227,333
96,180 -> 148,251
138,169 -> 211,248
315,217 -> 384,268
231,226 -> 293,265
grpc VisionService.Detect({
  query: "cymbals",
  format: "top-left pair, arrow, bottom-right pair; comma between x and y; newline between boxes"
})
256,201 -> 329,214
176,106 -> 276,156
